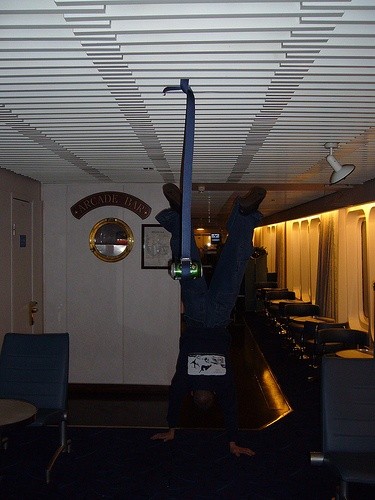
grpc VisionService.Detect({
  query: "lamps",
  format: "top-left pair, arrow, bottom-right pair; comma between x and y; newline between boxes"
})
325,143 -> 356,184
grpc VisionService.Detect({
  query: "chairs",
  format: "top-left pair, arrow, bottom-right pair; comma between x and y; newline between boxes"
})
255,287 -> 369,388
321,357 -> 375,500
0,332 -> 71,486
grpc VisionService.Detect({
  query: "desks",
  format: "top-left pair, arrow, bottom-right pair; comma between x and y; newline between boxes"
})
293,317 -> 334,360
335,350 -> 374,359
270,299 -> 307,335
0,399 -> 38,500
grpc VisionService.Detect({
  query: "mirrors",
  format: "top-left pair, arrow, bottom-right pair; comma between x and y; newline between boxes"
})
88,217 -> 135,263
